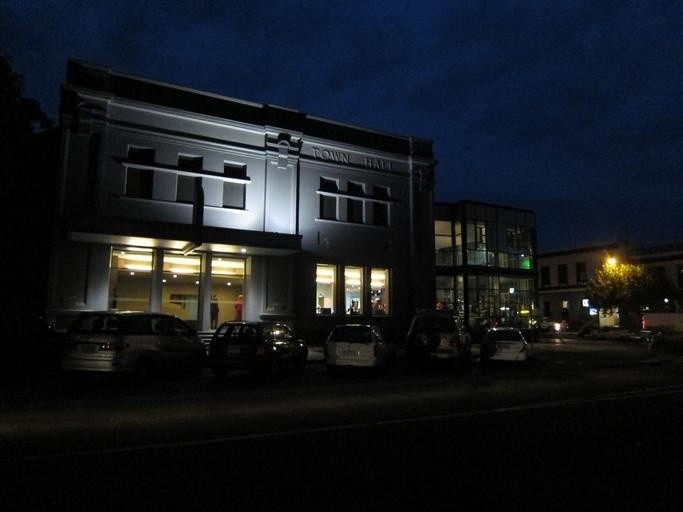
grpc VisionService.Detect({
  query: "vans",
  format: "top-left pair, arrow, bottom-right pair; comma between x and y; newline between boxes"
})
52,309 -> 208,397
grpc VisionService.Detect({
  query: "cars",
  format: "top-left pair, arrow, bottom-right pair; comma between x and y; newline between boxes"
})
208,319 -> 312,381
479,327 -> 528,366
541,321 -> 562,332
406,313 -> 472,372
323,324 -> 398,372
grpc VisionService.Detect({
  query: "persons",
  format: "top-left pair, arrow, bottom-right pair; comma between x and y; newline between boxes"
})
210,294 -> 219,329
350,300 -> 359,314
374,298 -> 386,314
471,300 -> 479,316
234,295 -> 243,320
436,298 -> 454,312
